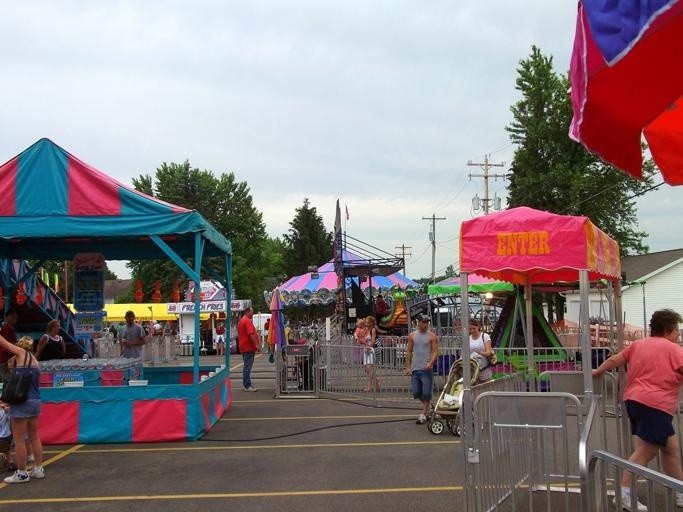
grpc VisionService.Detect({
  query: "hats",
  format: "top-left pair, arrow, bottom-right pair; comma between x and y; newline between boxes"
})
419,315 -> 431,321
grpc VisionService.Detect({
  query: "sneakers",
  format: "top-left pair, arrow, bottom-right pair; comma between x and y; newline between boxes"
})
613,492 -> 648,512
416,414 -> 430,424
676,491 -> 683,507
4,468 -> 45,483
244,386 -> 256,392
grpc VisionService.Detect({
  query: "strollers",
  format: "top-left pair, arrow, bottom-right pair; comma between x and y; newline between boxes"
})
426,359 -> 480,438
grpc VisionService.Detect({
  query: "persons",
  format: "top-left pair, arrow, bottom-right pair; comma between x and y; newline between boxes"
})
264,317 -> 319,341
460,317 -> 492,385
83,319 -> 178,344
590,308 -> 683,512
117,311 -> 148,359
354,316 -> 379,393
35,319 -> 65,361
404,314 -> 439,424
237,306 -> 260,392
0,308 -> 44,484
215,322 -> 225,356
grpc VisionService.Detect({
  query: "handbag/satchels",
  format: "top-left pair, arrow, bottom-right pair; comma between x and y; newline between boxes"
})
0,373 -> 32,404
487,346 -> 497,366
373,336 -> 383,355
